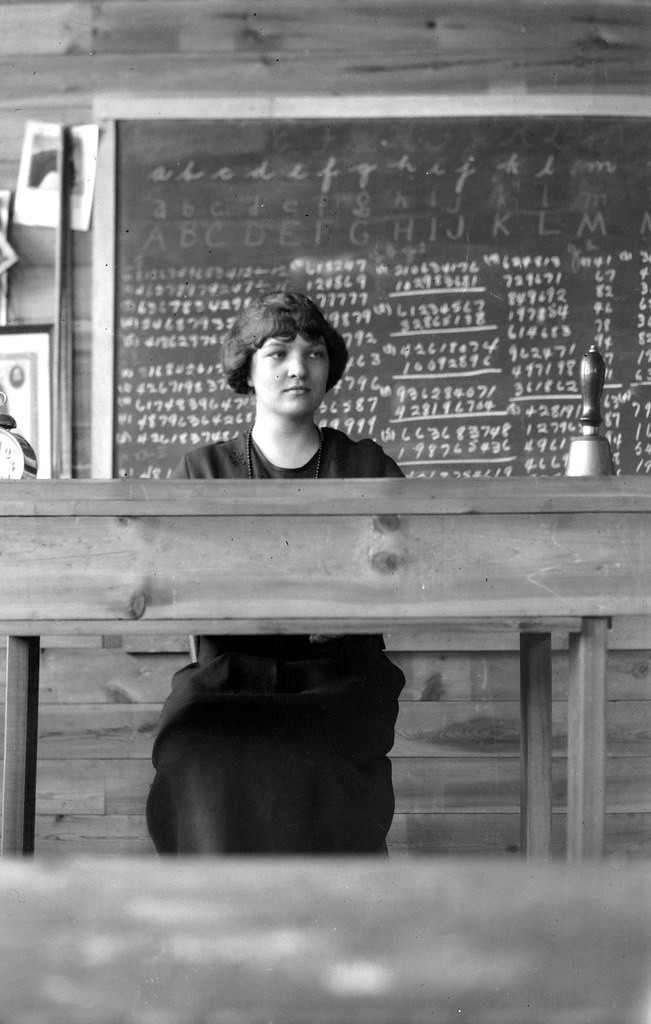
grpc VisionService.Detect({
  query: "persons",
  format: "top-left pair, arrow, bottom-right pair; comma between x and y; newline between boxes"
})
145,291 -> 406,860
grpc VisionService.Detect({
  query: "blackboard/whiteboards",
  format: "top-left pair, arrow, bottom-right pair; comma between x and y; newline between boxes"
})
90,94 -> 651,480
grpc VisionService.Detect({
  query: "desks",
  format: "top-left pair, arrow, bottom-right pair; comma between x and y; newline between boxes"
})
0,475 -> 650,859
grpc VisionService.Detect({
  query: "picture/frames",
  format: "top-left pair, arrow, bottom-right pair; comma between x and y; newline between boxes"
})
2,325 -> 56,481
